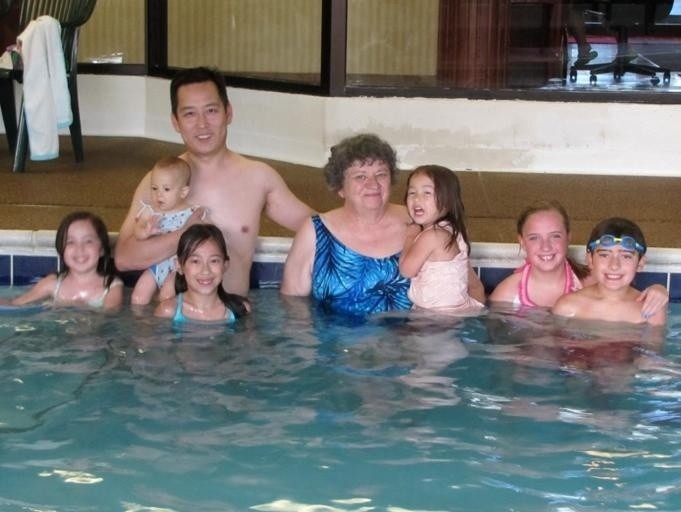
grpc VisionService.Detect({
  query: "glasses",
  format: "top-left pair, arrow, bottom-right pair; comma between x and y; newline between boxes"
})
588,234 -> 645,253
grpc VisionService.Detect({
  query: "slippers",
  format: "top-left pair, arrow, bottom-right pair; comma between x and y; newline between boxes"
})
574,51 -> 597,66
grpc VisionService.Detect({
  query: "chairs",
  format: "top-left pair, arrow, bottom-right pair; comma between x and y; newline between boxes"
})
568,0 -> 677,90
0,1 -> 102,171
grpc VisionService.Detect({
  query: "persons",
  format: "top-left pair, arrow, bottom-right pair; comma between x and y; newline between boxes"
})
152,223 -> 253,325
113,66 -> 319,297
550,217 -> 667,324
487,199 -> 670,319
0,210 -> 126,314
280,133 -> 486,317
128,155 -> 213,305
564,1 -> 637,68
398,163 -> 488,319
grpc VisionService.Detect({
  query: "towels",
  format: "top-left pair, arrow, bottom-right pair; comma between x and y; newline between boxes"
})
13,13 -> 78,164
